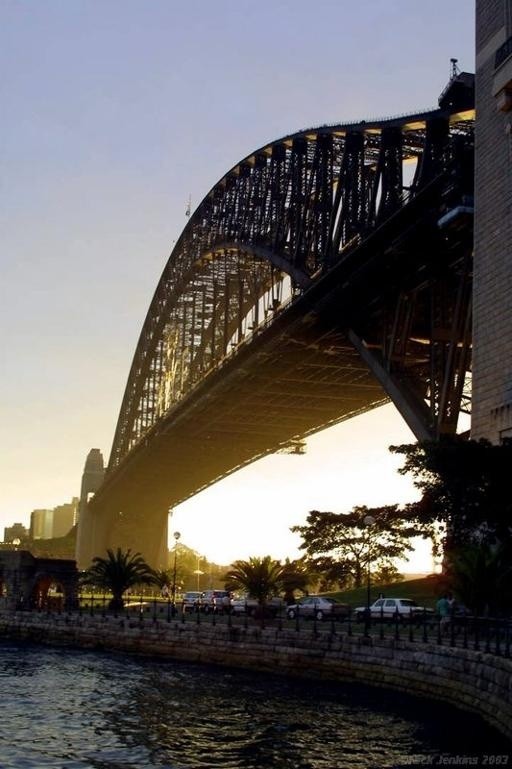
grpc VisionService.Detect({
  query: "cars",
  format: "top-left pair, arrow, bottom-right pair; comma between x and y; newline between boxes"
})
182,589 -> 437,627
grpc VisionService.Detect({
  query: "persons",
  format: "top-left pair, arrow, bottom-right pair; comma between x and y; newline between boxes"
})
446,592 -> 457,611
434,591 -> 453,640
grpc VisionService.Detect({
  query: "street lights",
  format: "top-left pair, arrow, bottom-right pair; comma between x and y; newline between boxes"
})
196,555 -> 204,591
171,531 -> 182,618
361,515 -> 377,629
